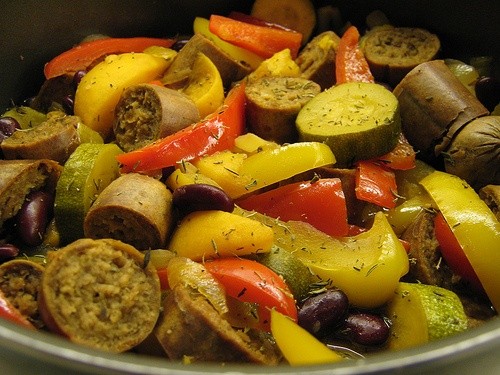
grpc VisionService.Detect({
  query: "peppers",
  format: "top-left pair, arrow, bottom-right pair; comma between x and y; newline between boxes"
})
44,13 -> 500,367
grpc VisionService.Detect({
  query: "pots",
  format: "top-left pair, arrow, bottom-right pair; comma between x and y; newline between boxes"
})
0,0 -> 500,375
